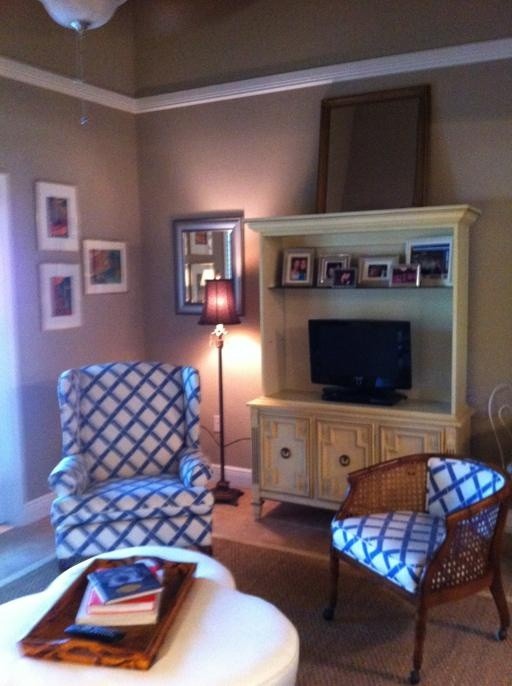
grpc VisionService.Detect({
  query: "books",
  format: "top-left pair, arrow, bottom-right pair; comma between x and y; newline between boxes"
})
74,563 -> 165,628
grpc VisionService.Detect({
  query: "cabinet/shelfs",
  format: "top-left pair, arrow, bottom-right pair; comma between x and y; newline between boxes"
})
245,202 -> 481,526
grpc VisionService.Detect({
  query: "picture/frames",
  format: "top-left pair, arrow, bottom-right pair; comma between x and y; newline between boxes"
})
333,268 -> 357,288
405,238 -> 452,285
171,217 -> 244,316
282,247 -> 314,286
390,264 -> 420,286
81,237 -> 128,296
35,180 -> 79,251
39,262 -> 82,330
357,254 -> 398,285
316,254 -> 351,288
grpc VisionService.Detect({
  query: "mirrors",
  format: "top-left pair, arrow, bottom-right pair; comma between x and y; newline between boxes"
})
316,82 -> 428,211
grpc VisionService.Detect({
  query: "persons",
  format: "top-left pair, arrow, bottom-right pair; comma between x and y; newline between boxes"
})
290,258 -> 306,279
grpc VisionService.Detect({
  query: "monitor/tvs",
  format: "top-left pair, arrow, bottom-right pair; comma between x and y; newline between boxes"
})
307,318 -> 412,406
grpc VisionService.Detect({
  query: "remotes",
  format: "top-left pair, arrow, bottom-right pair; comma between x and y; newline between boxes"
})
63,623 -> 127,643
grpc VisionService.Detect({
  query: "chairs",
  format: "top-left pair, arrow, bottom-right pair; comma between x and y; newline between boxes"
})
322,451 -> 512,685
488,383 -> 512,534
46,362 -> 216,567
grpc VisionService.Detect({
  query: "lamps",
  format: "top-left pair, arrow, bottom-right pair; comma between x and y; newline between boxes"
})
43,1 -> 126,126
198,280 -> 244,507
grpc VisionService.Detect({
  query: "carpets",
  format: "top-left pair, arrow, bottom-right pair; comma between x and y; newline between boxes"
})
0,538 -> 512,686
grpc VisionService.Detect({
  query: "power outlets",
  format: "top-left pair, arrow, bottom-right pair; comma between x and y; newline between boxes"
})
213,415 -> 221,432
